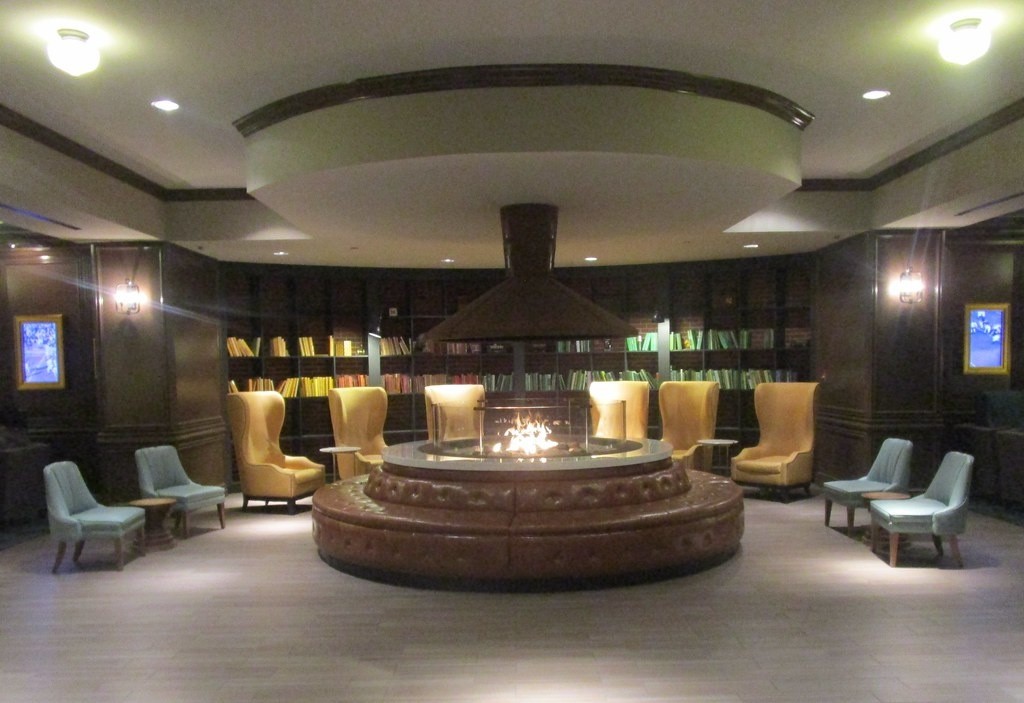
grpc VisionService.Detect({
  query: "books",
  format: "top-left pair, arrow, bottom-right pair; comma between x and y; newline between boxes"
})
329,336 -> 352,356
382,373 -> 412,393
669,332 -> 682,350
619,369 -> 659,389
576,339 -> 593,352
270,337 -> 287,356
414,373 -> 514,392
245,377 -> 299,397
337,374 -> 369,388
228,379 -> 238,393
226,337 -> 261,357
413,342 -> 505,352
626,332 -> 658,351
301,377 -> 334,397
380,336 -> 411,355
687,329 -> 750,350
557,341 -> 571,352
525,368 -> 615,391
671,368 -> 798,390
763,329 -> 774,349
299,337 -> 328,356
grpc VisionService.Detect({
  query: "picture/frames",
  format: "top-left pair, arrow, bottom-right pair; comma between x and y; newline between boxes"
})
15,313 -> 64,390
963,302 -> 1012,374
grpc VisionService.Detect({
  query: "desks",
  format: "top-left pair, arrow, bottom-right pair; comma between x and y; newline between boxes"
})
699,438 -> 738,475
132,498 -> 176,552
864,492 -> 911,549
320,446 -> 362,482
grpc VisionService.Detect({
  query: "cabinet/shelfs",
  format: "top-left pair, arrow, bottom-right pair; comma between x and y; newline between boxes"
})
223,316 -> 810,495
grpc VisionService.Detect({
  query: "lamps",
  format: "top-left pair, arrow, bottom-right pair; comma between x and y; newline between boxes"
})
114,279 -> 141,316
898,262 -> 925,304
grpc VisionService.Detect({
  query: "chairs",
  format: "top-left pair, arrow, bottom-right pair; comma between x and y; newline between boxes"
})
426,383 -> 484,441
655,381 -> 719,468
586,379 -> 648,441
868,451 -> 974,568
134,445 -> 226,537
326,386 -> 389,479
42,461 -> 145,571
822,438 -> 913,537
228,391 -> 324,512
731,382 -> 822,502
